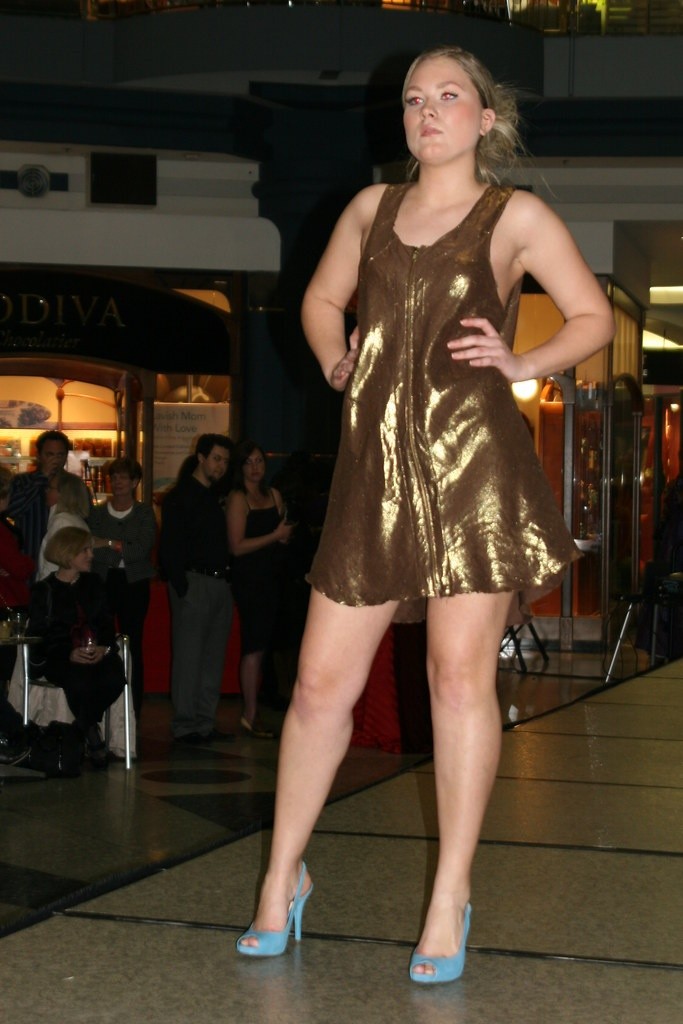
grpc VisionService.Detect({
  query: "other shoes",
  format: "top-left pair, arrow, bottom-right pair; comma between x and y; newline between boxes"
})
88,728 -> 109,768
240,716 -> 275,739
68,720 -> 87,767
181,727 -> 236,745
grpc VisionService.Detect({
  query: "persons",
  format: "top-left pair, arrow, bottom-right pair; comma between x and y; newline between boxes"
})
236,48 -> 615,983
0,433 -> 308,777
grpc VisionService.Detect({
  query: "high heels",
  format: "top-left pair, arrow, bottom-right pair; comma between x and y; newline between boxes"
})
409,902 -> 472,983
237,862 -> 313,957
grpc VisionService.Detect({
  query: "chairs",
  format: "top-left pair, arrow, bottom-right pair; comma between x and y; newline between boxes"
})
19,618 -> 131,770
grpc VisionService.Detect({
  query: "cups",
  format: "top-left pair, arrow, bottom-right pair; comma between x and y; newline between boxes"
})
80,635 -> 96,656
10,613 -> 27,637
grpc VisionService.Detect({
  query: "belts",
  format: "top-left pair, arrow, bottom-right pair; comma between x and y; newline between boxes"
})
190,569 -> 225,578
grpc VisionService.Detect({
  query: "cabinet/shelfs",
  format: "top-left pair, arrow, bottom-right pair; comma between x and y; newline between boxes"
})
0,422 -> 118,499
